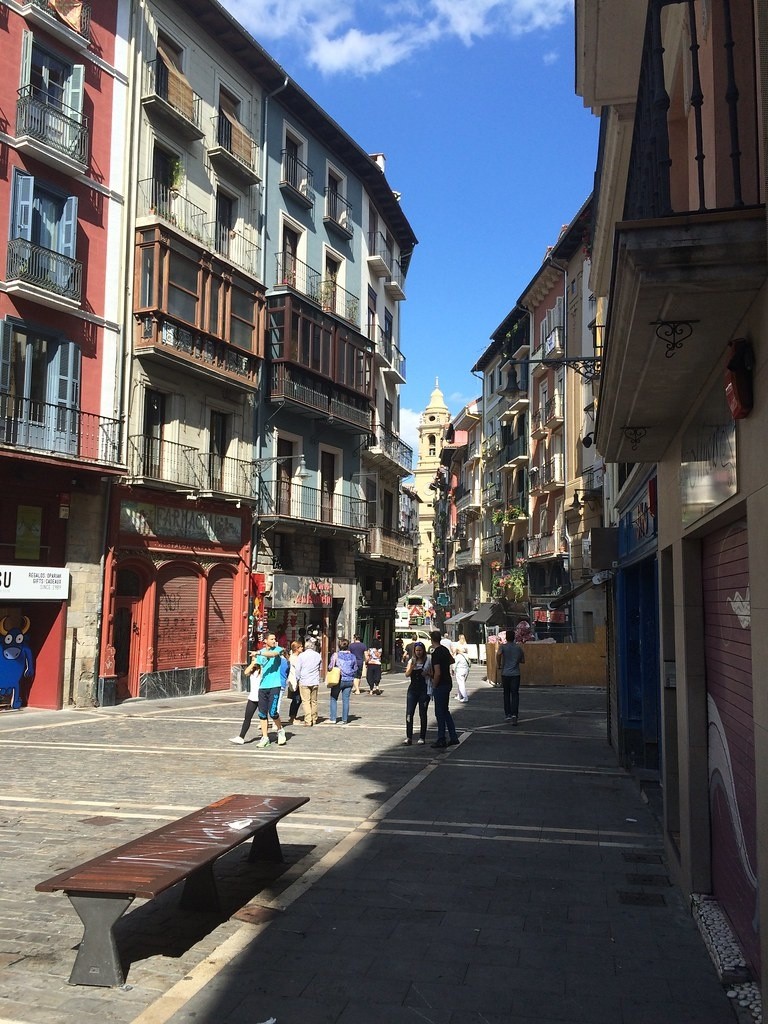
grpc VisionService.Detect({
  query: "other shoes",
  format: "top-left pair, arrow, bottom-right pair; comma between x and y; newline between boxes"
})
323,719 -> 337,723
502,714 -> 511,721
460,698 -> 468,703
402,738 -> 412,746
289,718 -> 301,724
417,738 -> 425,745
256,737 -> 270,748
451,694 -> 461,700
300,720 -> 313,727
337,718 -> 347,725
355,691 -> 360,695
376,686 -> 381,695
430,739 -> 447,748
369,690 -> 373,695
512,715 -> 518,727
277,725 -> 286,744
312,718 -> 316,724
351,686 -> 356,691
272,721 -> 278,732
446,738 -> 460,747
258,726 -> 262,730
228,736 -> 244,744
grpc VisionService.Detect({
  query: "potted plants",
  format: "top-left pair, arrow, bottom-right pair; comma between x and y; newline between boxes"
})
150,206 -> 169,220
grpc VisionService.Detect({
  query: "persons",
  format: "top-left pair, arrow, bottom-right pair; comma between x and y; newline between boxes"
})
396,635 -> 403,662
452,644 -> 471,703
324,639 -> 358,724
457,634 -> 467,649
440,633 -> 453,654
403,634 -> 417,663
348,634 -> 369,695
228,631 -> 286,748
402,642 -> 434,746
430,631 -> 460,747
274,624 -> 287,647
366,638 -> 382,695
287,640 -> 322,726
497,631 -> 525,726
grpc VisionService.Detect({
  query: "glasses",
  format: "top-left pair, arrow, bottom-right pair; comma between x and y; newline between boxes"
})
414,642 -> 423,646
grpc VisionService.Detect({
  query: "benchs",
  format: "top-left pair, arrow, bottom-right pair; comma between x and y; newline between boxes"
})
34,794 -> 315,991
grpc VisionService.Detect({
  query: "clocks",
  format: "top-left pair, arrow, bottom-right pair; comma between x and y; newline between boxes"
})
423,482 -> 436,496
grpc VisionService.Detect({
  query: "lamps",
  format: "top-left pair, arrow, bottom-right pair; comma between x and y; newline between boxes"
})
497,355 -> 603,399
569,489 -> 603,512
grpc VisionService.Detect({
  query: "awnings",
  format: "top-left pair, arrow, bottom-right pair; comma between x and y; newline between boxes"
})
470,603 -> 501,624
444,611 -> 476,624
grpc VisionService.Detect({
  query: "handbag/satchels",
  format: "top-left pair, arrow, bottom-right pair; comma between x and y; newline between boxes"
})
327,652 -> 340,688
288,685 -> 300,700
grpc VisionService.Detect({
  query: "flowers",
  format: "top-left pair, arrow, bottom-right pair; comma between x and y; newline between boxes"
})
491,560 -> 503,574
493,504 -> 522,515
515,556 -> 527,567
498,573 -> 513,588
